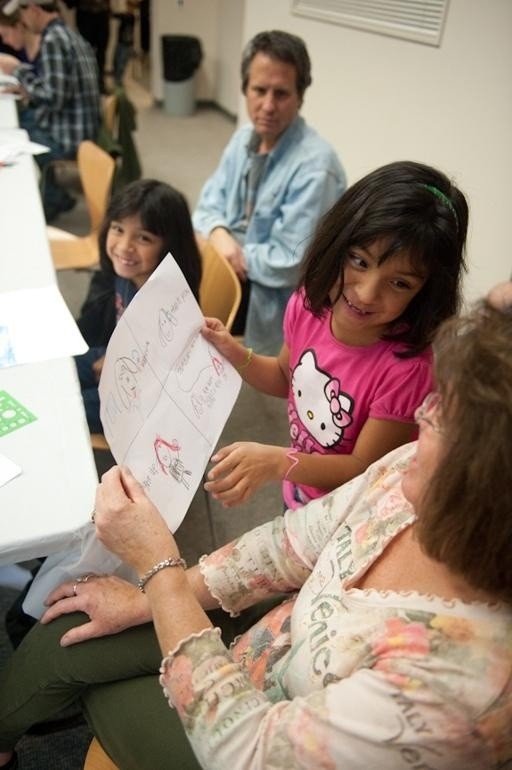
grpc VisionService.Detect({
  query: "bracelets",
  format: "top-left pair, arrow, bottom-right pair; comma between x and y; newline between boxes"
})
285,447 -> 299,480
139,556 -> 187,594
236,348 -> 253,374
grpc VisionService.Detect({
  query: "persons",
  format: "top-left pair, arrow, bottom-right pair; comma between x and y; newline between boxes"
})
0,277 -> 512,770
1,0 -> 138,224
202,162 -> 468,509
191,31 -> 347,356
71,179 -> 202,434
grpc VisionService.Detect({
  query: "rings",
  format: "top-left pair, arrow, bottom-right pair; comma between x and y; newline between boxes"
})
76,576 -> 83,582
73,583 -> 78,596
91,509 -> 97,525
83,575 -> 90,582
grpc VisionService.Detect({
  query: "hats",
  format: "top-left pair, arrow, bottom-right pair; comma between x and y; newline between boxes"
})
1,1 -> 57,16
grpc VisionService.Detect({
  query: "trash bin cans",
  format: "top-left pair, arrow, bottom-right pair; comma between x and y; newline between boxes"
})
161,34 -> 202,115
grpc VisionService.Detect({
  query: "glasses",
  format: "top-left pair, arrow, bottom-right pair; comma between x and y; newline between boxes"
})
415,391 -> 457,446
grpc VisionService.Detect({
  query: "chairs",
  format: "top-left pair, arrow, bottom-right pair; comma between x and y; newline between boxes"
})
90,242 -> 242,448
40,93 -> 122,196
46,140 -> 116,271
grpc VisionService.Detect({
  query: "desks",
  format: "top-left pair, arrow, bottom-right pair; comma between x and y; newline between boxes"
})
1,96 -> 101,588
